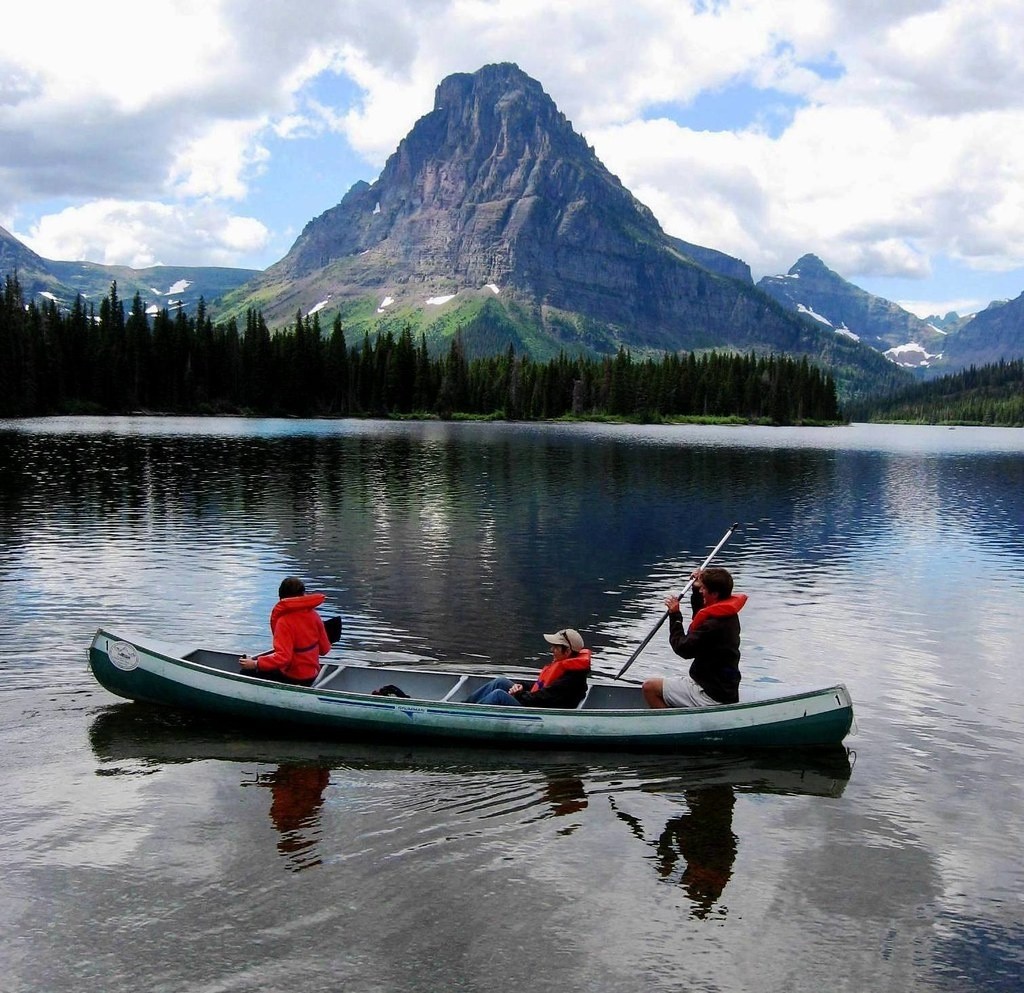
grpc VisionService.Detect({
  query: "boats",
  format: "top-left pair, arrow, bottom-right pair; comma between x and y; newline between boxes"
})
86,627 -> 856,749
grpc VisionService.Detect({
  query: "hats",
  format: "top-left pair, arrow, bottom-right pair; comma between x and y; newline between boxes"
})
543,629 -> 584,652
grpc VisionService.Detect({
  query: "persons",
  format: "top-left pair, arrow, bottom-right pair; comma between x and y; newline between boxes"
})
642,568 -> 748,709
239,577 -> 332,687
461,628 -> 590,709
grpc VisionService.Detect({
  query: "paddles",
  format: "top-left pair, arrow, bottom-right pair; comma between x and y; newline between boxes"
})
614,521 -> 740,680
241,616 -> 342,662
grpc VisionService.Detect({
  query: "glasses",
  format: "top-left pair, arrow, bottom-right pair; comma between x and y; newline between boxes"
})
561,630 -> 572,649
